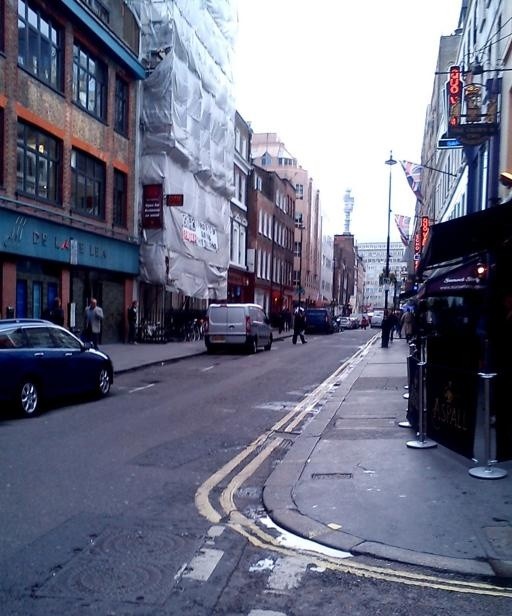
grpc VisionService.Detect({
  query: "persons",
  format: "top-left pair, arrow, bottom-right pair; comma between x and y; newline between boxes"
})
83,297 -> 103,349
361,317 -> 366,330
291,309 -> 308,344
401,308 -> 415,338
127,300 -> 138,344
51,297 -> 64,326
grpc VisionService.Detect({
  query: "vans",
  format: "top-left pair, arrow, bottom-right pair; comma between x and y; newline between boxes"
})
203,303 -> 273,354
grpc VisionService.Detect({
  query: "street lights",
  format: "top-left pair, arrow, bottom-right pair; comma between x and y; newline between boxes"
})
391,270 -> 399,331
332,256 -> 349,306
298,220 -> 306,310
381,149 -> 398,347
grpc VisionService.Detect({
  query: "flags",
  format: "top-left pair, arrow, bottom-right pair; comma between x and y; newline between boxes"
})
393,214 -> 411,246
400,160 -> 424,203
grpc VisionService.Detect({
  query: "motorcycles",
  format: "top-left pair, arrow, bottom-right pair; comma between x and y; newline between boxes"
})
331,314 -> 343,332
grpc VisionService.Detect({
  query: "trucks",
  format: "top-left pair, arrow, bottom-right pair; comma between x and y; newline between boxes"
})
303,308 -> 334,335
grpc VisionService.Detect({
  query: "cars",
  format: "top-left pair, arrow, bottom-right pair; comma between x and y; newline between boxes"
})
338,305 -> 400,330
293,306 -> 305,315
1,319 -> 114,419
